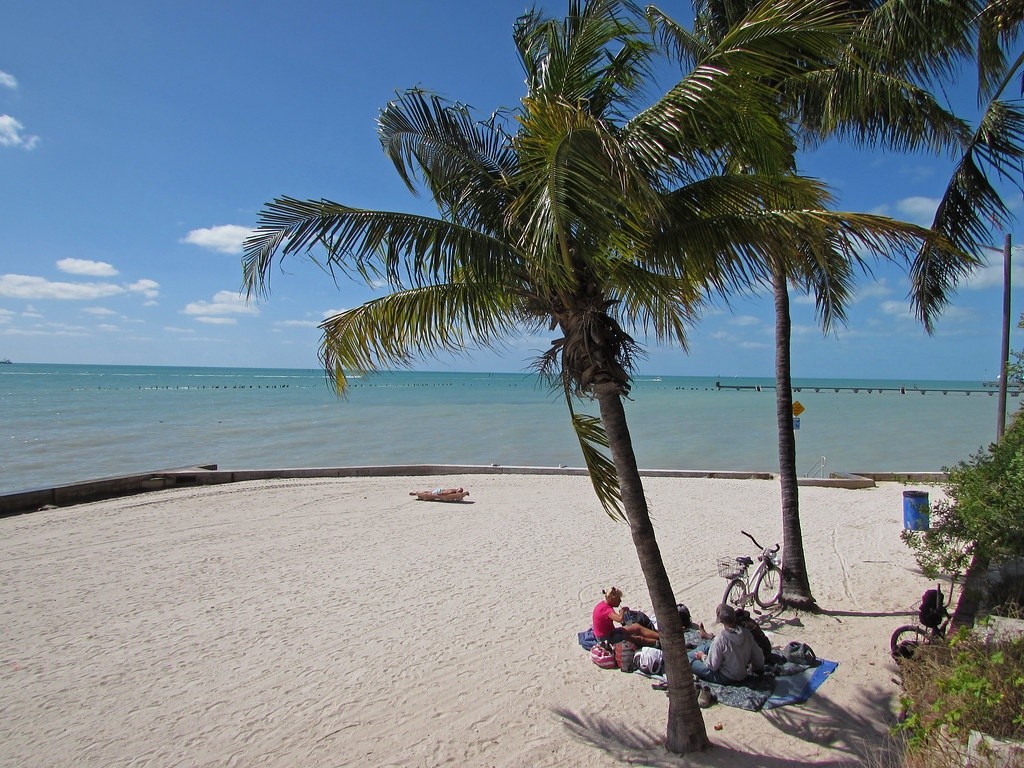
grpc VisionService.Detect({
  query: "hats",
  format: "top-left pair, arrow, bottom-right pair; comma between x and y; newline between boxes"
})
716,604 -> 735,624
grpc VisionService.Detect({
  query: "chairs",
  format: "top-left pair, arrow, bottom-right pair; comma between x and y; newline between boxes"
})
417,490 -> 469,500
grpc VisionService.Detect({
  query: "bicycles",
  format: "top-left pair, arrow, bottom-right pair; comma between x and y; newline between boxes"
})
716,530 -> 785,612
890,571 -> 962,665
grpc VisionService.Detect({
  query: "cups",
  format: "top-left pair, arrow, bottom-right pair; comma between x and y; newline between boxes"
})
624,610 -> 631,622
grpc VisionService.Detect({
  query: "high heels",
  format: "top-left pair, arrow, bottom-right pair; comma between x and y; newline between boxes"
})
651,682 -> 668,690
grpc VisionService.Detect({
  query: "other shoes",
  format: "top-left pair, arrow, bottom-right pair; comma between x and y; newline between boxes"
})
694,685 -> 700,695
697,686 -> 710,707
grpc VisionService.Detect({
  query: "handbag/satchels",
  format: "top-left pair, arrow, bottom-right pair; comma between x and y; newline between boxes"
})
590,642 -> 616,668
784,641 -> 816,665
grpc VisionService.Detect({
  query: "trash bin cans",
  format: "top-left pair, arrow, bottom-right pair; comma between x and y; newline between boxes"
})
902,491 -> 929,531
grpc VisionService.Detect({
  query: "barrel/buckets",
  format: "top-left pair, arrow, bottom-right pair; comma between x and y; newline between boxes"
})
903,491 -> 929,531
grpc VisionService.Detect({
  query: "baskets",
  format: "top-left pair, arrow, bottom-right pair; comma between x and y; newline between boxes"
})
909,599 -> 932,629
716,556 -> 739,577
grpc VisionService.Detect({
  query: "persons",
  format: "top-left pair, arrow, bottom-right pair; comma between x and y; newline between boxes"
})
592,587 -> 660,645
688,603 -> 772,686
435,488 -> 463,495
